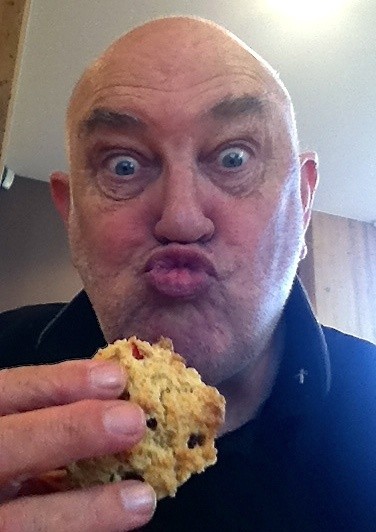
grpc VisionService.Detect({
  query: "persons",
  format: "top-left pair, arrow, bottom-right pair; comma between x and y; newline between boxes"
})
0,12 -> 376,530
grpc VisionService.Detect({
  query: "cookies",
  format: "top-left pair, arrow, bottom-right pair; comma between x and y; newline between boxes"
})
64,336 -> 226,500
